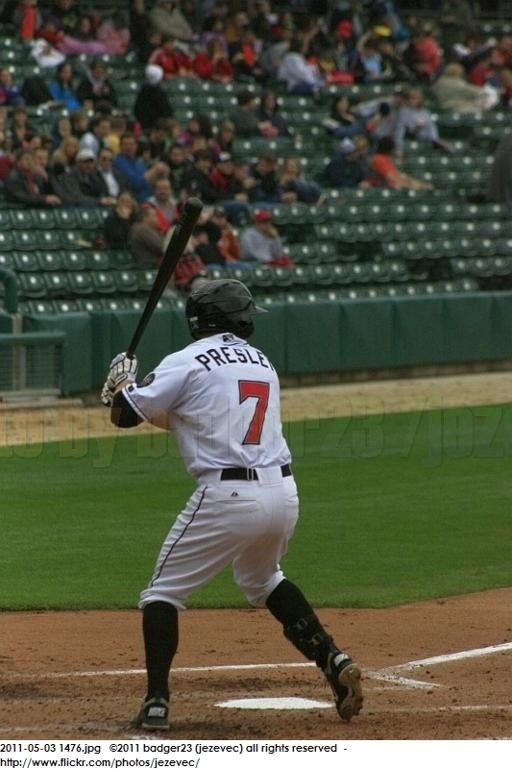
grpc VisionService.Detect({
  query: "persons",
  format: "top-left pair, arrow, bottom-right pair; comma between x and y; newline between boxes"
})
100,278 -> 366,730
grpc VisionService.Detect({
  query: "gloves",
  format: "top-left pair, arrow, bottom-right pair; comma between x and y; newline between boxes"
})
100,349 -> 141,408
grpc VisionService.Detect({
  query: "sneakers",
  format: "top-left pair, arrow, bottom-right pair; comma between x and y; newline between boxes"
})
325,652 -> 365,720
128,695 -> 170,730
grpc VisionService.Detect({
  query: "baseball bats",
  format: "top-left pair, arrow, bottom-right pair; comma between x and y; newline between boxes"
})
125,195 -> 204,357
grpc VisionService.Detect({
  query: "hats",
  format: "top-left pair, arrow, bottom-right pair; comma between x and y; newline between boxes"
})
144,63 -> 164,84
256,209 -> 273,223
76,148 -> 96,161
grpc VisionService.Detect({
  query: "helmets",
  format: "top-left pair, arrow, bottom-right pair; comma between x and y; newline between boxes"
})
185,279 -> 270,341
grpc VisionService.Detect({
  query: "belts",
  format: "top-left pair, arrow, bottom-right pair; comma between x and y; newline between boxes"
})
219,463 -> 292,483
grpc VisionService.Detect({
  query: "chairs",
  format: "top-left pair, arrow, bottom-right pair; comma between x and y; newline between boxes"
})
0,20 -> 511,316
